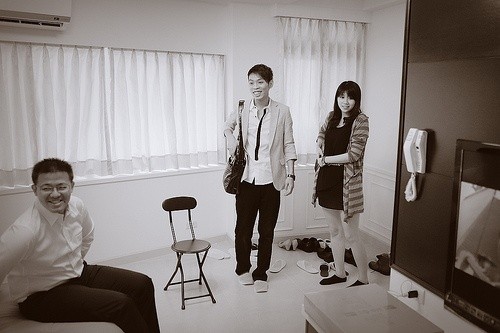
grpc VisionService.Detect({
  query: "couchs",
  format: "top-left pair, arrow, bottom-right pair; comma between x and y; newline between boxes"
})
0,281 -> 124,333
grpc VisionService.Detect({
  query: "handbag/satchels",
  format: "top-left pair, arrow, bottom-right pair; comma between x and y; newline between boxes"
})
223,147 -> 246,194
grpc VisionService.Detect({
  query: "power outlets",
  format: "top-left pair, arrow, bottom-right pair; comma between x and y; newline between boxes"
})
411,284 -> 425,305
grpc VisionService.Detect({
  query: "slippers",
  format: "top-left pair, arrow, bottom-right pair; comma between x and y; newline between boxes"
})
326,262 -> 349,276
270,259 -> 286,273
206,249 -> 224,259
319,262 -> 330,277
236,272 -> 253,285
348,280 -> 368,288
297,260 -> 319,274
212,249 -> 230,259
319,273 -> 346,285
254,280 -> 268,292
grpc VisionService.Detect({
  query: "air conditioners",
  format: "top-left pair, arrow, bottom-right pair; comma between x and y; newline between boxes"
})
0,0 -> 72,31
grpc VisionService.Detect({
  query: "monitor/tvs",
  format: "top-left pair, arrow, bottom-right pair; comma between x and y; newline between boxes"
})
444,137 -> 500,332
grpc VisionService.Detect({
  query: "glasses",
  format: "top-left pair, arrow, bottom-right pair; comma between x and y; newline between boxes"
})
36,181 -> 73,193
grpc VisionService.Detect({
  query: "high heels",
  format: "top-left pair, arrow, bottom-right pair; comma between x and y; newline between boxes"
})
291,239 -> 298,250
279,239 -> 291,251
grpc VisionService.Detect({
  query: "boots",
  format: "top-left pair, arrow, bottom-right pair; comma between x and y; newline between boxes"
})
368,253 -> 391,276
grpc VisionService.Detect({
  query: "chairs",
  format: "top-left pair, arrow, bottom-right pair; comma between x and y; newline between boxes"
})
162,196 -> 217,310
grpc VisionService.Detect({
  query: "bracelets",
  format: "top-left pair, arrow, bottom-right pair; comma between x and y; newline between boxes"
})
322,157 -> 327,165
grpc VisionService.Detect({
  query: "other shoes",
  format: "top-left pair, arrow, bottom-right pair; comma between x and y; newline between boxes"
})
318,238 -> 325,248
317,247 -> 334,263
310,237 -> 321,252
325,239 -> 332,248
298,238 -> 312,254
343,248 -> 357,267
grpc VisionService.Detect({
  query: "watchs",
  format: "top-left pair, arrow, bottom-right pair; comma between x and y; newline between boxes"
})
287,175 -> 295,181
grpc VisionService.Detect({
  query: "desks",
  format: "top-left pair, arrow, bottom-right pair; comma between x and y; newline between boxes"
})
301,283 -> 444,333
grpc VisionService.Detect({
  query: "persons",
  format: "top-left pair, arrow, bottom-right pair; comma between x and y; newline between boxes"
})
223,64 -> 297,293
0,158 -> 160,333
310,81 -> 369,288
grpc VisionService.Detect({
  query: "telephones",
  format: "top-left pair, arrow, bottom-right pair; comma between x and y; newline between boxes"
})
402,128 -> 429,174
